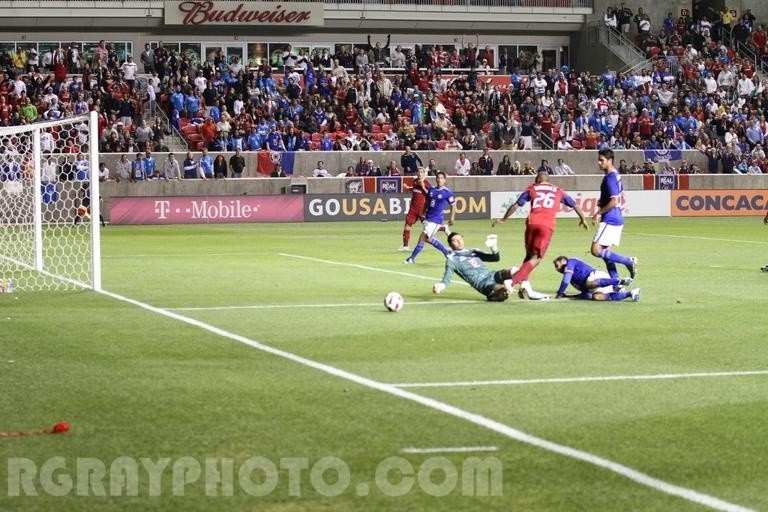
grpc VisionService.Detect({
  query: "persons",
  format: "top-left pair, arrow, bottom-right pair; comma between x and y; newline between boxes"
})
759,194 -> 768,272
550,255 -> 642,303
400,171 -> 456,264
492,170 -> 589,300
432,231 -> 545,301
589,148 -> 638,292
396,165 -> 431,254
70,180 -> 105,227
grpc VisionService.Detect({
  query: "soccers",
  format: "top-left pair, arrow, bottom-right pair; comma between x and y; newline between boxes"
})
385,291 -> 405,312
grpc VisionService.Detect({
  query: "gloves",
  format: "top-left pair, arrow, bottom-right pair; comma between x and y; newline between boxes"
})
433,283 -> 446,294
485,235 -> 498,252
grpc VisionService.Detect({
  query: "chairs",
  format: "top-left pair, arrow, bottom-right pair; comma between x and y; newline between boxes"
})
546,118 -> 598,151
159,91 -> 500,154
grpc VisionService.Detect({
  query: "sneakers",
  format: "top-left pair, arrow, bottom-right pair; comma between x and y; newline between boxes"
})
760,263 -> 768,272
632,288 -> 640,302
443,224 -> 451,237
627,256 -> 638,279
397,245 -> 409,252
403,258 -> 416,264
487,288 -> 510,303
612,285 -> 625,293
625,277 -> 632,286
518,286 -> 526,300
504,279 -> 513,296
